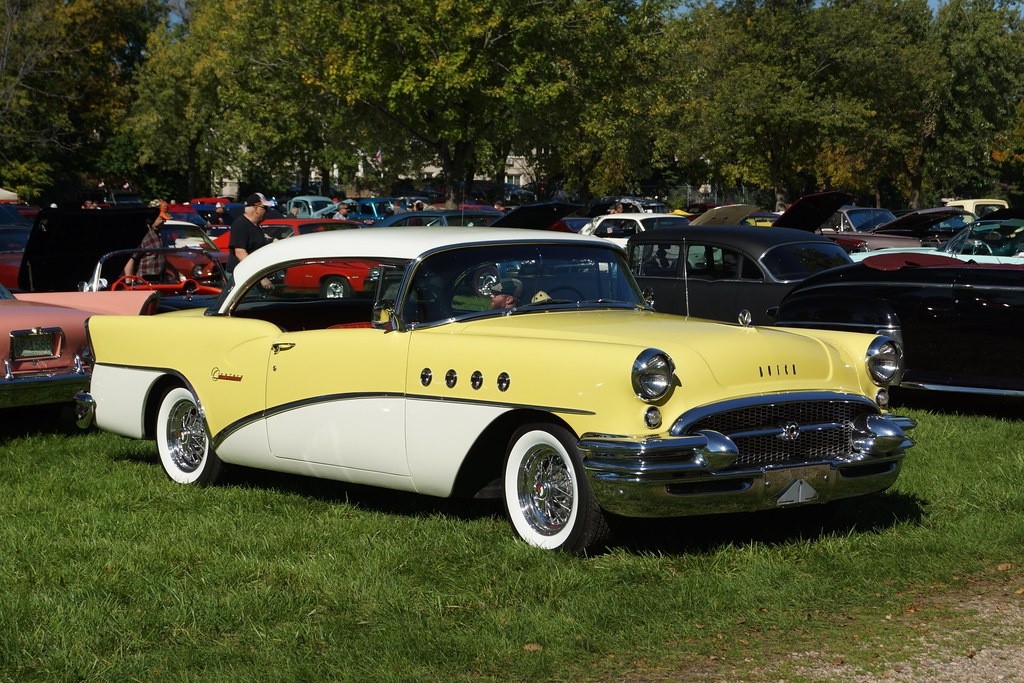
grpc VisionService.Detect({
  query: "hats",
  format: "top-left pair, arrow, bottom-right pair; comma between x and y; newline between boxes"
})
487,278 -> 524,299
244,192 -> 275,207
494,200 -> 503,205
216,202 -> 225,207
148,199 -> 173,220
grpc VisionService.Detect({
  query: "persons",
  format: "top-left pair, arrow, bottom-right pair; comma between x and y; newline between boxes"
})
125,198 -> 174,285
407,201 -> 429,213
80,200 -> 103,210
284,207 -> 300,219
493,200 -> 512,215
332,202 -> 350,220
207,201 -> 226,225
226,192 -> 275,298
487,277 -> 525,309
607,203 -> 624,214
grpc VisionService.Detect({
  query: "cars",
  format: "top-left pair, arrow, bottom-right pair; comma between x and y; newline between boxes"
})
0,187 -> 161,409
687,203 -> 736,221
849,219 -> 1024,271
577,214 -> 721,271
78,179 -> 576,327
624,226 -> 854,326
815,205 -> 975,254
600,196 -> 675,214
76,227 -> 918,560
939,199 -> 1009,228
737,213 -> 781,227
772,252 -> 1024,403
548,217 -> 617,233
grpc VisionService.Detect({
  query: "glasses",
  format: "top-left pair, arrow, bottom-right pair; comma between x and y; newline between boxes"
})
339,208 -> 347,210
491,290 -> 508,296
